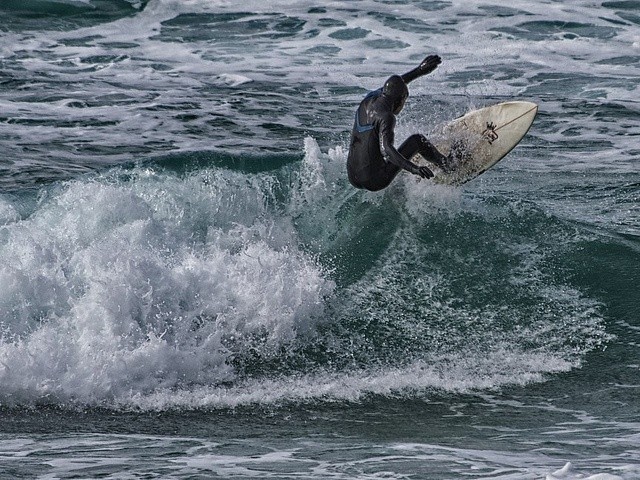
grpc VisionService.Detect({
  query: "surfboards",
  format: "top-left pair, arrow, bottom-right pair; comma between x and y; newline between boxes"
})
412,101 -> 538,189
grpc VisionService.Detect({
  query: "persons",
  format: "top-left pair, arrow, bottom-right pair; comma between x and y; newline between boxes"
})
347,54 -> 455,192
